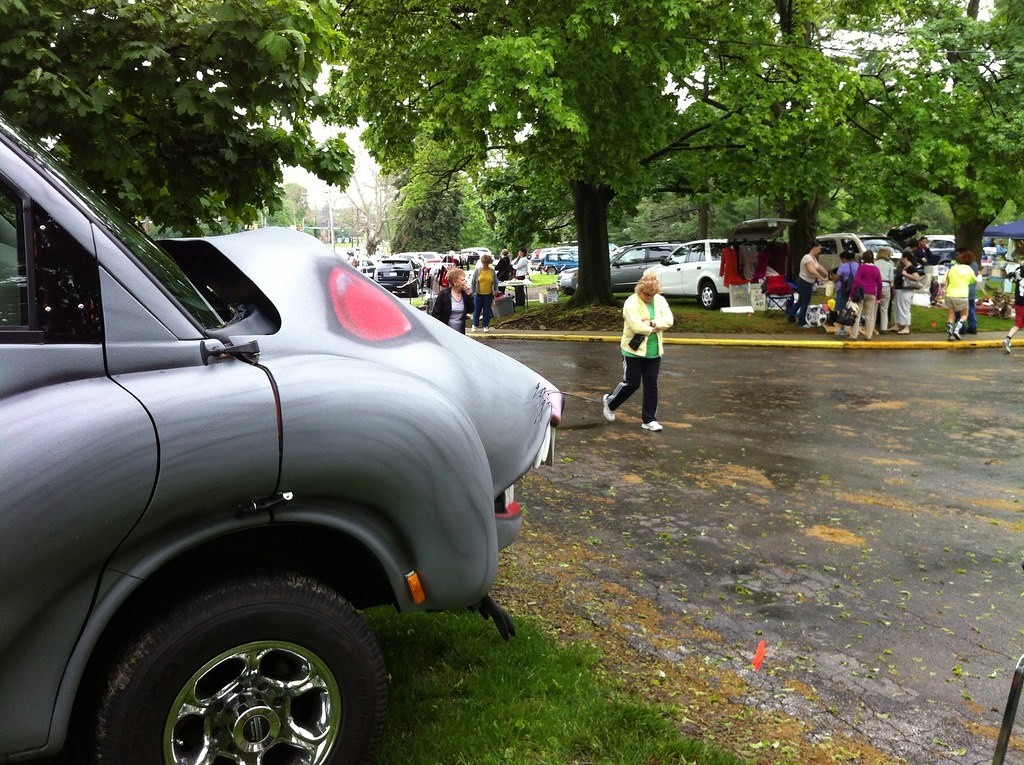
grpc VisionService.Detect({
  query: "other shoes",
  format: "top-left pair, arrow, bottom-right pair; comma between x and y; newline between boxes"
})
803,324 -> 811,329
847,336 -> 855,340
787,316 -> 799,323
967,329 -> 978,335
897,329 -> 910,334
959,329 -> 967,334
864,336 -> 870,341
880,330 -> 887,334
472,324 -> 477,331
483,325 -> 489,333
1002,338 -> 1011,353
887,326 -> 898,332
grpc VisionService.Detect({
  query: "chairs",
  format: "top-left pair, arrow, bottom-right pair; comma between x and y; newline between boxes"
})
763,273 -> 794,319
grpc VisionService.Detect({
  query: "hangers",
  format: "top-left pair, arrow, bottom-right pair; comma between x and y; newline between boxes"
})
729,237 -> 785,249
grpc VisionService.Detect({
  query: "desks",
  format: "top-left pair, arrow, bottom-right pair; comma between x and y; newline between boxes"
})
497,281 -> 528,309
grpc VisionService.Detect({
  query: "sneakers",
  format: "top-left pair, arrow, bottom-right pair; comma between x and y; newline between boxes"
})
641,421 -> 663,432
603,394 -> 616,422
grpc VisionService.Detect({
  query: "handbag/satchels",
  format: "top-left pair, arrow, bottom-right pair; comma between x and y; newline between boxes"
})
490,291 -> 516,317
902,272 -> 924,290
852,285 -> 864,303
841,273 -> 855,294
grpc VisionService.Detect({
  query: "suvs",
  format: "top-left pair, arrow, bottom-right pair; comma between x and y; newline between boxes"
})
555,242 -> 685,297
641,217 -> 798,310
920,234 -> 956,273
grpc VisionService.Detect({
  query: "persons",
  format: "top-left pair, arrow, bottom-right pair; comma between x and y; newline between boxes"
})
1002,257 -> 1024,353
603,273 -> 673,431
830,237 -> 1008,341
458,248 -> 529,332
432,268 -> 475,335
786,241 -> 829,327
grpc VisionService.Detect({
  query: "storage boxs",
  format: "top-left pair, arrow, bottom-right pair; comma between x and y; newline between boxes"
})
537,285 -> 558,303
923,246 -> 1020,293
491,295 -> 514,318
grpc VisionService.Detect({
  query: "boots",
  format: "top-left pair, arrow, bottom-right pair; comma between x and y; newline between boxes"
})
953,318 -> 965,341
946,322 -> 955,341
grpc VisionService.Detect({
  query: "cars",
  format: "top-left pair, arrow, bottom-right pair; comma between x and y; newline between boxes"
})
347,246 -> 496,297
526,244 -> 620,275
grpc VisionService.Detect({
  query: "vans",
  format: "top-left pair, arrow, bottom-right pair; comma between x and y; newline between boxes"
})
816,233 -> 905,274
0,117 -> 564,765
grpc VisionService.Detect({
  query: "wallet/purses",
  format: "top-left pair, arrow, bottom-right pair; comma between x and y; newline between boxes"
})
628,330 -> 646,351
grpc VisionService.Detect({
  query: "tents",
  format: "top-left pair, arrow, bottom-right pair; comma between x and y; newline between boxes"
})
983,220 -> 1024,248
